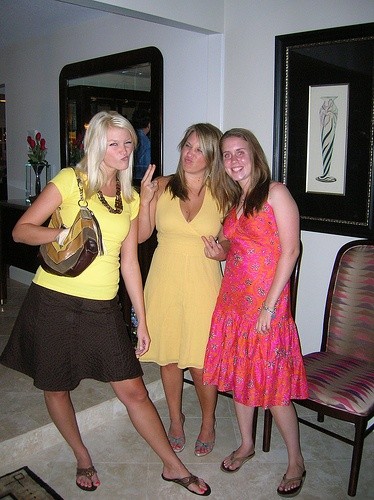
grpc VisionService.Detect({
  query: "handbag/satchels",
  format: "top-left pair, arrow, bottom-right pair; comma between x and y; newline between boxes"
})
36,166 -> 104,278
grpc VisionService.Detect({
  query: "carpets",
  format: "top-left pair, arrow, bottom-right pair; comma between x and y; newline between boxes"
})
0,466 -> 64,500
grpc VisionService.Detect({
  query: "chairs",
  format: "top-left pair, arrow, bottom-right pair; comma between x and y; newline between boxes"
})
261,239 -> 374,497
179,238 -> 304,448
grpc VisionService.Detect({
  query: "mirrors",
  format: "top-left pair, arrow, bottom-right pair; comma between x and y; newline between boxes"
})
58,46 -> 163,189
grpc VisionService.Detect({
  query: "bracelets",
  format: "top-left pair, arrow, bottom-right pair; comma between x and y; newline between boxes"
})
263,302 -> 276,314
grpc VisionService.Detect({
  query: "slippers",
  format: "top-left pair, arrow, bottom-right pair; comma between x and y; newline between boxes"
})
76,466 -> 97,492
161,473 -> 211,496
276,469 -> 306,498
168,432 -> 185,453
220,451 -> 256,473
194,439 -> 215,456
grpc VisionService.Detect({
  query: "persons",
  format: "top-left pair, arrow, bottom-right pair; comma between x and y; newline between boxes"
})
1,112 -> 212,496
203,128 -> 307,497
136,123 -> 241,458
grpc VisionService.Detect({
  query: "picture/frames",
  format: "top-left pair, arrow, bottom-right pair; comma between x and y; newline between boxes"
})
270,22 -> 374,239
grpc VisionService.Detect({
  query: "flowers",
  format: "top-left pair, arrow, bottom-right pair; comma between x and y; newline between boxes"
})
25,132 -> 48,164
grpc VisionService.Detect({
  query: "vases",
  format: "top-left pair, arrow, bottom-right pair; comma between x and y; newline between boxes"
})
25,161 -> 51,206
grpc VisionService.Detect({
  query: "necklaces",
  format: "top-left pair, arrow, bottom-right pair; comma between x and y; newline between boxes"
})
180,187 -> 205,223
94,173 -> 124,214
